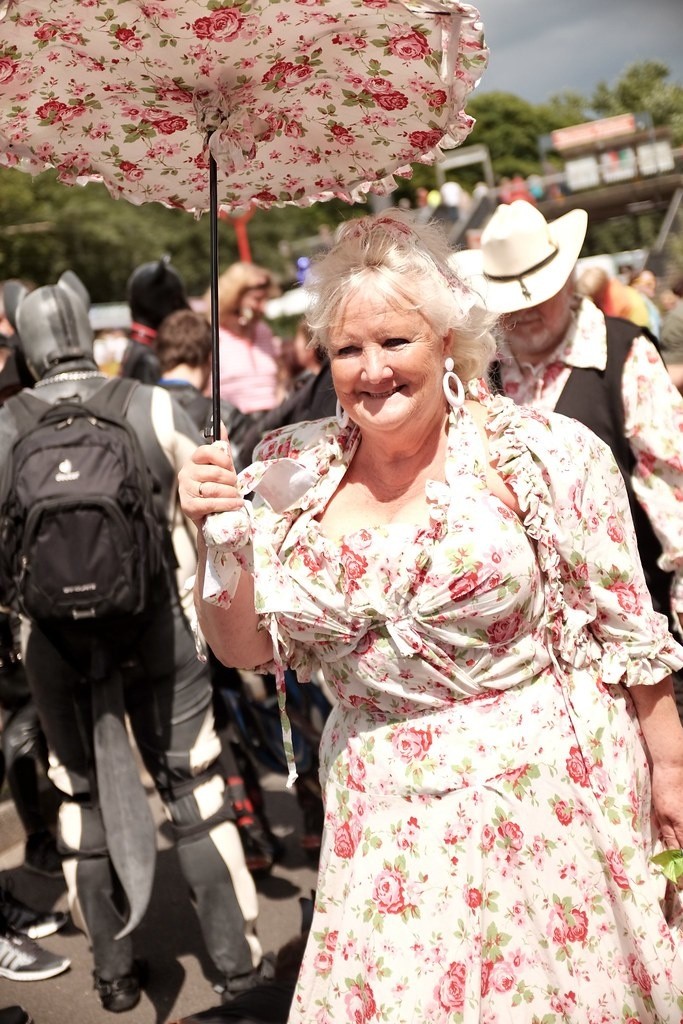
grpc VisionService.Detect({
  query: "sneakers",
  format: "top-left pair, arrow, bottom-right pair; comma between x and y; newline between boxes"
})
0,920 -> 72,981
0,877 -> 70,939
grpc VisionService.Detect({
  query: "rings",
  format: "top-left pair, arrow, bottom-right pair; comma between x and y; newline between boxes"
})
199,483 -> 204,497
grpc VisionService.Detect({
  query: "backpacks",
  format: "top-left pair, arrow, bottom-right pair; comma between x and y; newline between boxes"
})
0,377 -> 170,632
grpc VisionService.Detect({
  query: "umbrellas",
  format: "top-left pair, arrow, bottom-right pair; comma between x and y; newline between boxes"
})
0,1 -> 488,606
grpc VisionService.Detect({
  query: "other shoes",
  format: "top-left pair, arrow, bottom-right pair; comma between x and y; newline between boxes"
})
92,954 -> 148,1012
25,835 -> 63,877
213,957 -> 302,1009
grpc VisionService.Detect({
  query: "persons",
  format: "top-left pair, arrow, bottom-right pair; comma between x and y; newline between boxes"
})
448,196 -> 683,650
171,210 -> 683,1024
0,260 -> 341,1024
634,270 -> 662,340
578,268 -> 647,335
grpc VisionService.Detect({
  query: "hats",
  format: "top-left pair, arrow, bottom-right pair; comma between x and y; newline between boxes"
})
445,200 -> 588,313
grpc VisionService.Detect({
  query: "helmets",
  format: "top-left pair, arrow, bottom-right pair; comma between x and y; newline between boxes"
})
4,270 -> 94,378
